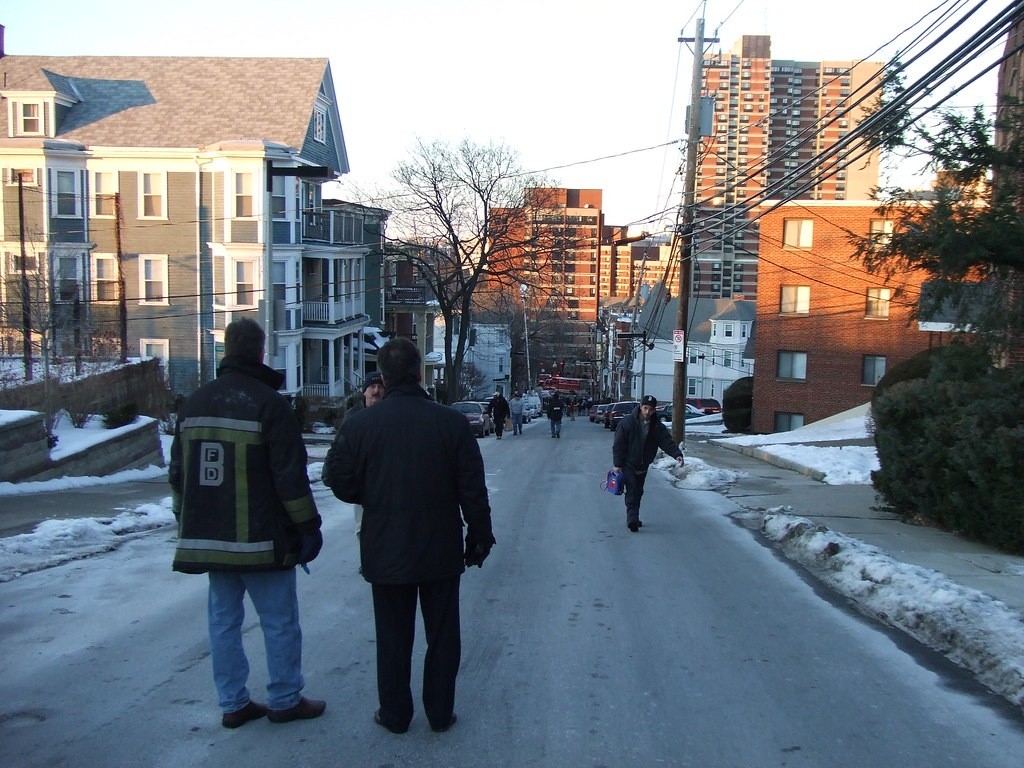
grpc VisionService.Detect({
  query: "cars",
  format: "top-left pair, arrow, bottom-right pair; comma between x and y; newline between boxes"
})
450,401 -> 490,438
590,398 -> 721,431
479,374 -> 592,434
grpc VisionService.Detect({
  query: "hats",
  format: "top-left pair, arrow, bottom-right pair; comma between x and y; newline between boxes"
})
492,392 -> 500,397
642,395 -> 657,407
362,371 -> 383,394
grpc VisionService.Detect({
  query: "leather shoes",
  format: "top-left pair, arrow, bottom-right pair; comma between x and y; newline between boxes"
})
267,698 -> 326,723
222,700 -> 268,729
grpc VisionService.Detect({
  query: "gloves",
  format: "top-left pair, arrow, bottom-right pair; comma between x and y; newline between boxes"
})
297,530 -> 323,564
464,529 -> 497,568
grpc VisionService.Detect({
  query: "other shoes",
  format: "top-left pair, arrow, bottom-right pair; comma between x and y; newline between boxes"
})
556,432 -> 560,437
519,427 -> 522,434
628,520 -> 642,531
552,435 -> 555,438
431,712 -> 458,731
514,433 -> 517,435
374,709 -> 408,734
496,435 -> 501,440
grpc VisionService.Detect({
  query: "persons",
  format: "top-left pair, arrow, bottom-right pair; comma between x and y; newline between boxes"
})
508,394 -> 523,435
486,392 -> 510,440
321,373 -> 387,575
612,395 -> 684,533
167,316 -> 324,729
547,394 -> 565,439
328,335 -> 496,735
577,402 -> 582,416
570,402 -> 576,421
566,405 -> 570,417
